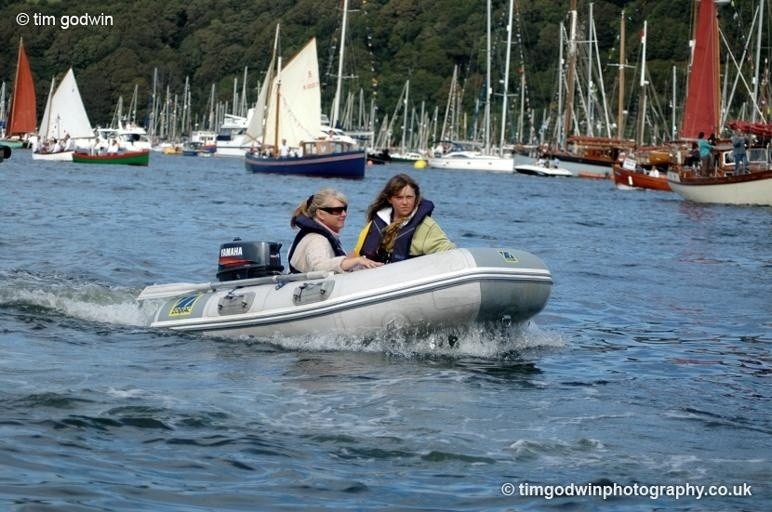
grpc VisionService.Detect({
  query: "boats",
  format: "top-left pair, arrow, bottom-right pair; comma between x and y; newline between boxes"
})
130,238 -> 552,350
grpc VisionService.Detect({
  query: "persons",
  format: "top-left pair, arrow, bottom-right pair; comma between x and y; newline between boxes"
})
295,140 -> 304,158
278,138 -> 292,158
91,138 -> 102,149
107,139 -> 120,153
62,133 -> 74,152
347,173 -> 457,271
33,135 -> 48,154
286,187 -> 385,276
697,127 -> 752,177
682,141 -> 700,167
97,146 -> 105,155
325,129 -> 338,150
536,154 -> 560,170
49,138 -> 62,153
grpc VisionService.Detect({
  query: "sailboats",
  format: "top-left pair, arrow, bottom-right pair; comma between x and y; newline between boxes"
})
0,0 -> 772,207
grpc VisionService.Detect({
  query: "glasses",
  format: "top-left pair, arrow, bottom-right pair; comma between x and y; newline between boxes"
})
319,205 -> 347,215
